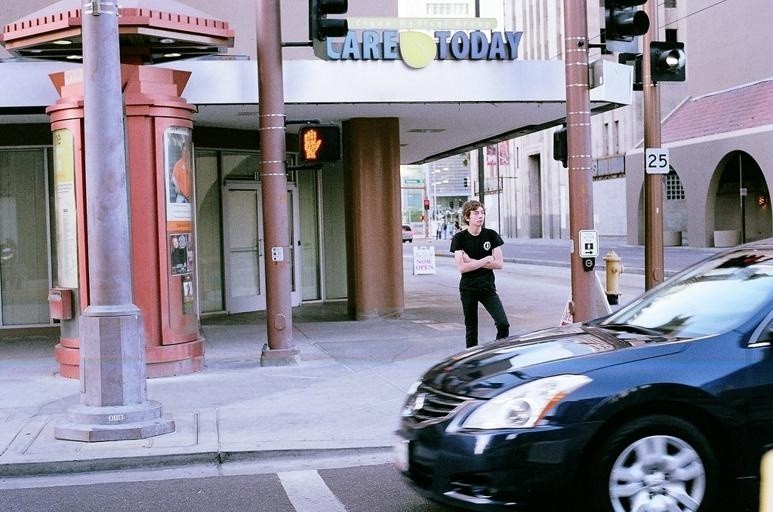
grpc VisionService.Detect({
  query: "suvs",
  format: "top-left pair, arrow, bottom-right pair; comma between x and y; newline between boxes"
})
402,224 -> 413,243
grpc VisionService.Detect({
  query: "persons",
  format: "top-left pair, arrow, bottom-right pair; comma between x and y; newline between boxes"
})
436,222 -> 459,241
448,200 -> 509,348
172,143 -> 190,202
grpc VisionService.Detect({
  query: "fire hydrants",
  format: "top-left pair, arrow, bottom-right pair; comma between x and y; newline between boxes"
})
602,247 -> 623,305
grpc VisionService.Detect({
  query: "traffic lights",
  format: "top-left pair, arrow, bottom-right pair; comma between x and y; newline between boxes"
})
600,0 -> 650,56
424,200 -> 429,209
649,41 -> 687,83
298,125 -> 342,160
305,0 -> 350,40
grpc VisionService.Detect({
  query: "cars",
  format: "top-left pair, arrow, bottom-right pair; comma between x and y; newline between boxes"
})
389,236 -> 773,512
